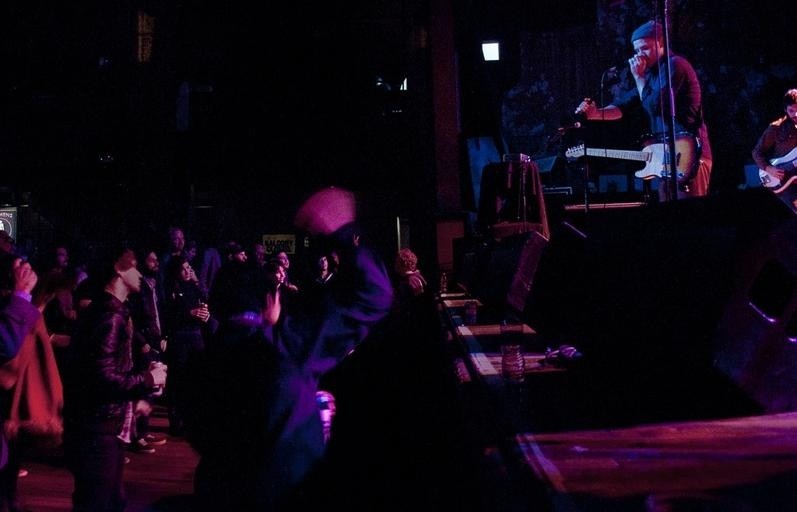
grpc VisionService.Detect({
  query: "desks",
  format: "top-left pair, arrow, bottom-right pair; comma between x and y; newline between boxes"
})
433,264 -> 729,512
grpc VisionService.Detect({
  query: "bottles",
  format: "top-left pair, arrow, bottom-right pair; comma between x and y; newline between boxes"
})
440,271 -> 447,293
500,303 -> 524,379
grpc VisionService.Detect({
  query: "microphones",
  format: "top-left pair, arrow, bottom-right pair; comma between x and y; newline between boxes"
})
592,77 -> 623,97
612,52 -> 648,86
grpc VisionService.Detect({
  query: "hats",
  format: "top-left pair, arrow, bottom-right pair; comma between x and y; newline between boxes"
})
629,18 -> 665,43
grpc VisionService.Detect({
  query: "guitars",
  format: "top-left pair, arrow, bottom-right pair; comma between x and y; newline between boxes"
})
564,132 -> 701,186
758,148 -> 793,196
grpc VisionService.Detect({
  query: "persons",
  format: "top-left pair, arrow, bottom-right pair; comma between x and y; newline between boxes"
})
575,20 -> 715,203
751,88 -> 797,214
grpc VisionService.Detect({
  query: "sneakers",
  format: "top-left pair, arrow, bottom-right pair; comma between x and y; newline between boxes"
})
136,437 -> 157,454
144,430 -> 167,446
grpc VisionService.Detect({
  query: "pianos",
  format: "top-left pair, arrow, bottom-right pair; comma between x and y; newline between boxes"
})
532,155 -> 584,203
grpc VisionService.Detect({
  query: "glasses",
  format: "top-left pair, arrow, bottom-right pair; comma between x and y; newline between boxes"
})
181,264 -> 193,273
